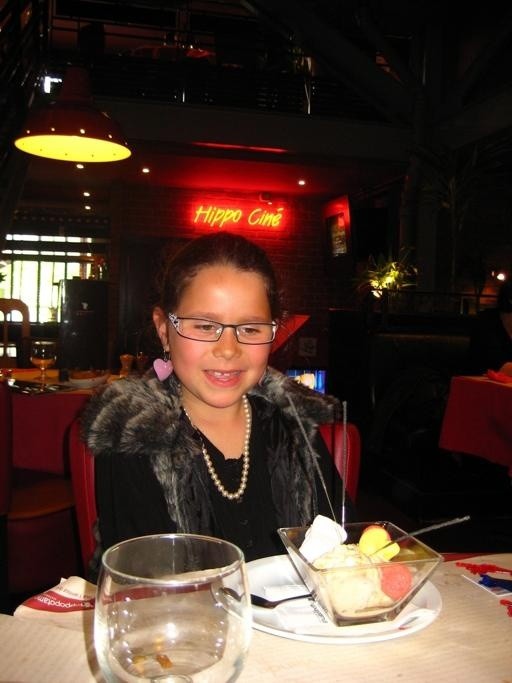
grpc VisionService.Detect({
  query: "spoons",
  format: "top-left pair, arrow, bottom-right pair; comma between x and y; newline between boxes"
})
223,583 -> 311,609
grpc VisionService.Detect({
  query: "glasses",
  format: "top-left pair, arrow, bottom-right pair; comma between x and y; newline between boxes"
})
166,311 -> 280,345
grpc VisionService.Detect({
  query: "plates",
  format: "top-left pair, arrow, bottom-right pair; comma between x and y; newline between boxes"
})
209,549 -> 449,648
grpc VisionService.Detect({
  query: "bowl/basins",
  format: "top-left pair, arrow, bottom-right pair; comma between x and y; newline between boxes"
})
68,369 -> 110,389
275,520 -> 444,624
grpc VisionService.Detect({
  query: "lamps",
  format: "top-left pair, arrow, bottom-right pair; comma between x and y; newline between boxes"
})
14,0 -> 133,163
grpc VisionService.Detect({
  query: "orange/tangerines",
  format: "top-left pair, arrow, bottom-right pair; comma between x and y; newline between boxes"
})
381,564 -> 412,601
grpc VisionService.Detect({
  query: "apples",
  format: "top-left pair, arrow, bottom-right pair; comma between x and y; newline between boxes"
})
357,525 -> 400,560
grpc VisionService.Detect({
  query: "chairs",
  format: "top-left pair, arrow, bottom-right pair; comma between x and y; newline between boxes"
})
69,418 -> 359,573
0,379 -> 84,583
0,295 -> 32,370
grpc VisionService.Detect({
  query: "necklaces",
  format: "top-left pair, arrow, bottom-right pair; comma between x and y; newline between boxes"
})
179,388 -> 253,501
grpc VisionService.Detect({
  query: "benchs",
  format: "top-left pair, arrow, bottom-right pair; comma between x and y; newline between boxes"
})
367,332 -> 473,477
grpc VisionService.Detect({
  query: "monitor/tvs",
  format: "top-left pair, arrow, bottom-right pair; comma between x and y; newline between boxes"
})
283,368 -> 327,395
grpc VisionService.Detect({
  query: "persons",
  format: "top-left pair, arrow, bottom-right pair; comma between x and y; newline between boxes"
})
467,276 -> 512,380
94,232 -> 356,584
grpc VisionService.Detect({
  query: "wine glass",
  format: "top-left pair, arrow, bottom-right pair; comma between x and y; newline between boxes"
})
29,340 -> 60,395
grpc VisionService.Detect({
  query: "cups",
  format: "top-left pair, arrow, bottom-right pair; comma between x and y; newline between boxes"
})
120,354 -> 148,378
91,533 -> 254,683
